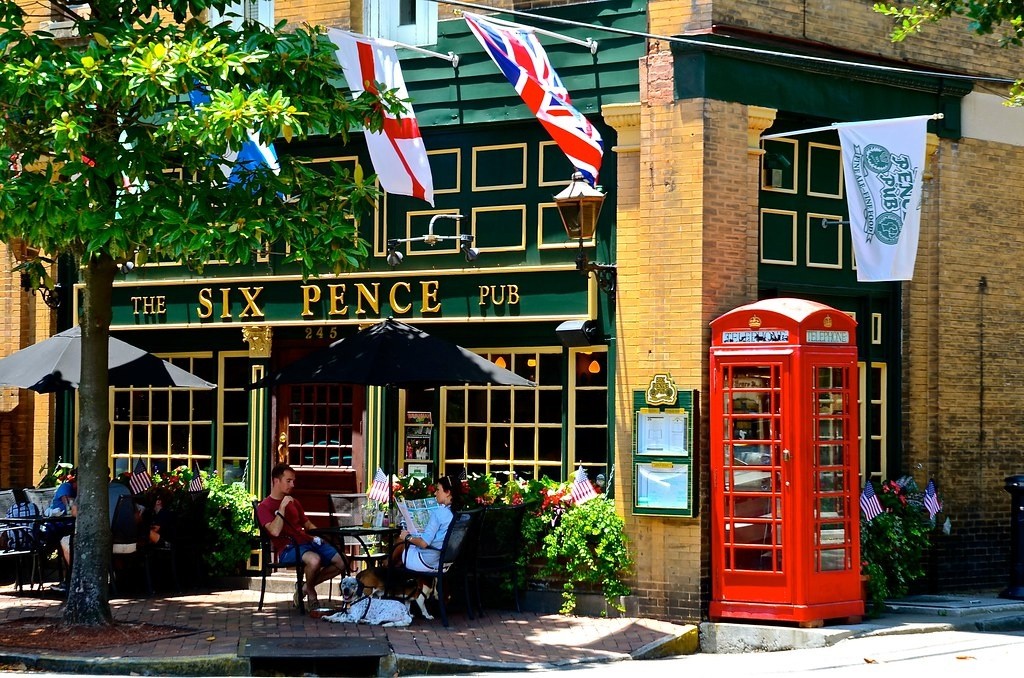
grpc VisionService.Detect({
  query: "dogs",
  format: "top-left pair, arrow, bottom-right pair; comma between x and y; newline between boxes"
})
321,576 -> 416,628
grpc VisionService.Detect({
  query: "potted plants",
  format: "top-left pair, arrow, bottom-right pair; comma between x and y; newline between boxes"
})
550,500 -> 635,619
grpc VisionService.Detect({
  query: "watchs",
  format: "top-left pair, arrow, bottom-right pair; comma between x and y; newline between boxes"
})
405,534 -> 412,541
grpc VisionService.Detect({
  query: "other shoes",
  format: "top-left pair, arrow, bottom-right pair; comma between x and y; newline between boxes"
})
308,599 -> 320,611
51,581 -> 67,591
293,590 -> 306,607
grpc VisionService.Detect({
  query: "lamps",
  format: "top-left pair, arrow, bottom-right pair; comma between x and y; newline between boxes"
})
385,234 -> 480,262
552,169 -> 616,304
11,239 -> 62,308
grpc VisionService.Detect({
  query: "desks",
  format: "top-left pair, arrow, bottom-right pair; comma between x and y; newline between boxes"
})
308,526 -> 404,606
0,514 -> 76,593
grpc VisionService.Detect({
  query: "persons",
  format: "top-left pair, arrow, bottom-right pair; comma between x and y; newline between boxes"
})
49,469 -> 174,599
409,417 -> 430,435
406,441 -> 427,460
383,475 -> 471,603
257,463 -> 346,611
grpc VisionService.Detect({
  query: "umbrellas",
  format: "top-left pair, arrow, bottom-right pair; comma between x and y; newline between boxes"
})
243,314 -> 537,592
0,324 -> 216,394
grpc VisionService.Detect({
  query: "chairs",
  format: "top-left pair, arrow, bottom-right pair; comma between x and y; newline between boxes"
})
408,507 -> 486,627
0,485 -> 210,599
253,499 -> 332,615
328,492 -> 401,601
442,504 -> 523,619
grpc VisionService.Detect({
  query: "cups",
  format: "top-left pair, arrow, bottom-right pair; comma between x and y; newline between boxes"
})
362,508 -> 372,527
388,514 -> 395,528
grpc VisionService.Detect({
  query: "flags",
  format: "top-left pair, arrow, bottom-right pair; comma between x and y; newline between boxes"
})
860,481 -> 883,522
369,468 -> 390,503
188,78 -> 283,199
571,467 -> 596,504
70,102 -> 156,220
924,482 -> 940,520
129,457 -> 152,494
327,30 -> 435,207
463,13 -> 604,190
839,120 -> 928,282
189,464 -> 203,492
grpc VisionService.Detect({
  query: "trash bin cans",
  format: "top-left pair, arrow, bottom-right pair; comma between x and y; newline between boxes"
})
998,475 -> 1024,601
238,636 -> 389,678
